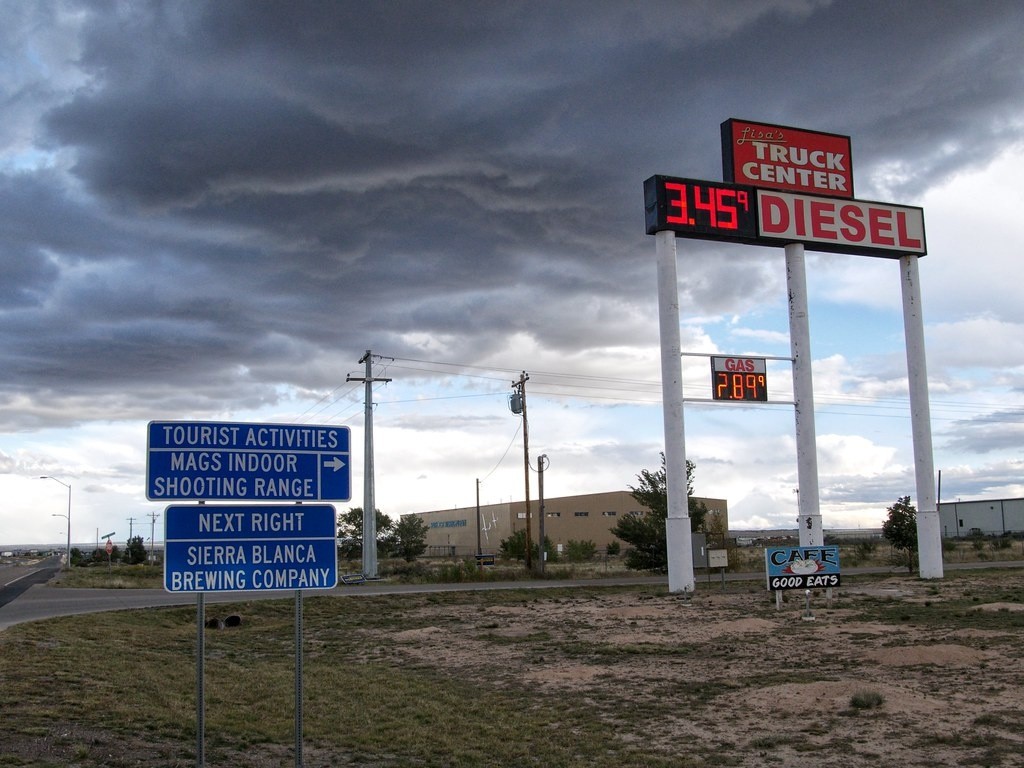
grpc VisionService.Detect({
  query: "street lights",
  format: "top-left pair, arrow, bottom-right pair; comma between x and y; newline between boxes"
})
40,476 -> 71,569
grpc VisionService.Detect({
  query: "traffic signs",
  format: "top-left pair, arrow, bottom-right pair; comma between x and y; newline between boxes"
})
164,504 -> 338,594
144,418 -> 351,504
